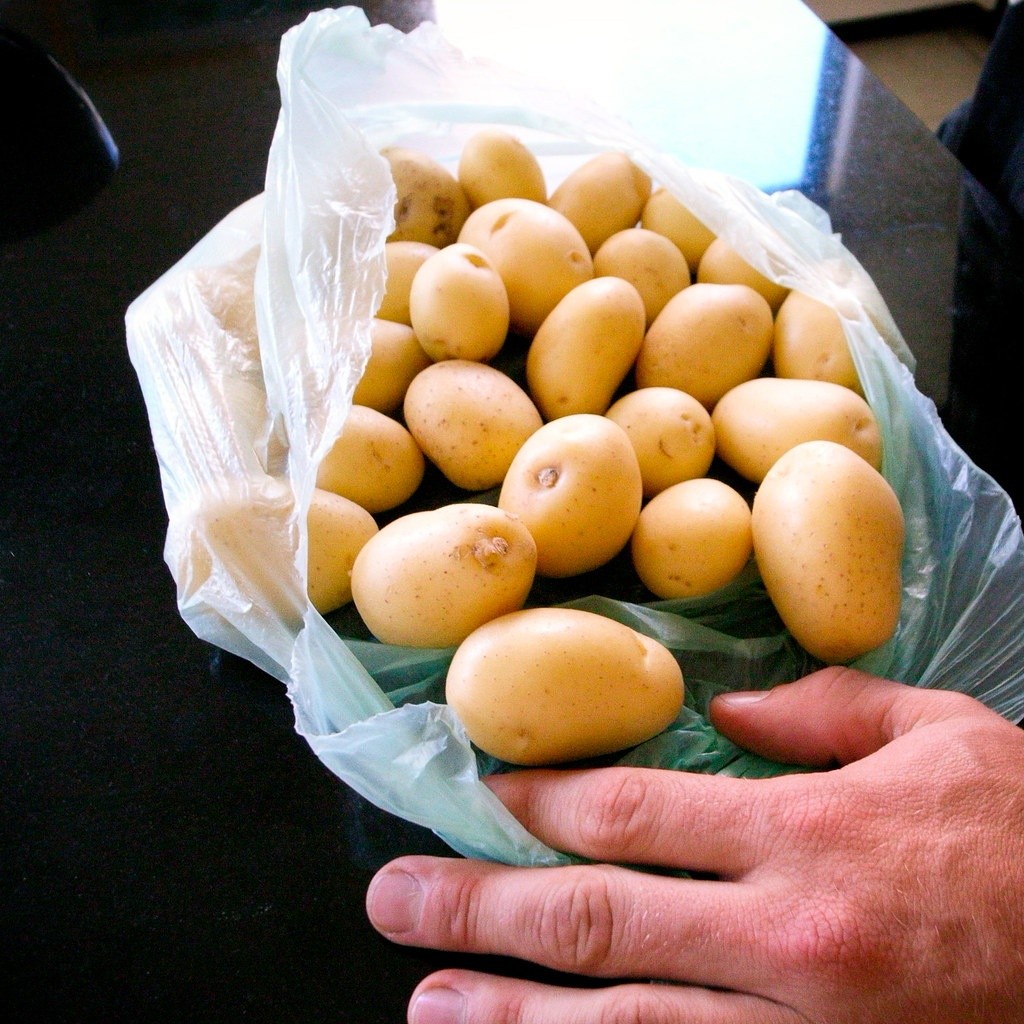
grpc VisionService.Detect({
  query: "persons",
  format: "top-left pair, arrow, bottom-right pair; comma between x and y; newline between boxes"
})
367,0 -> 1024,1023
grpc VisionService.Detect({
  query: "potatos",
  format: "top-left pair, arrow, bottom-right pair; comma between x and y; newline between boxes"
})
145,133 -> 905,763
